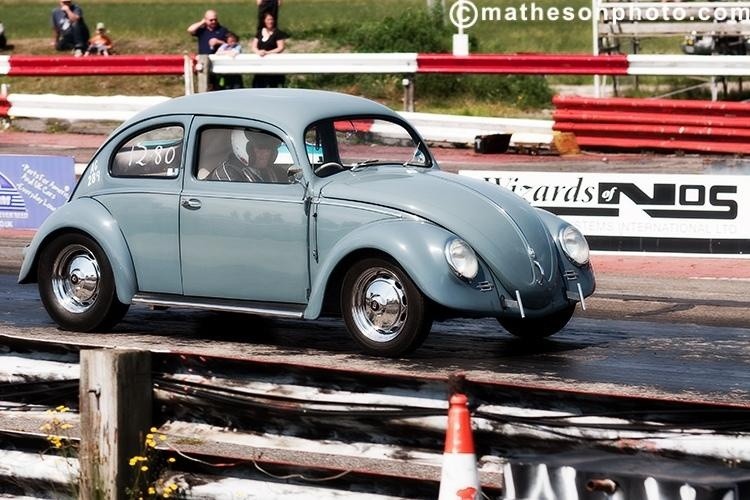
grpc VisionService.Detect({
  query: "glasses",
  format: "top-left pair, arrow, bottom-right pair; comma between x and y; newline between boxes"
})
206,17 -> 217,22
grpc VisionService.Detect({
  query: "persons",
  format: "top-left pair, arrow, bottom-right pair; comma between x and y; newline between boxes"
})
254,1 -> 281,38
213,31 -> 244,91
48,0 -> 89,57
185,8 -> 240,92
207,126 -> 296,184
251,11 -> 286,88
84,20 -> 115,57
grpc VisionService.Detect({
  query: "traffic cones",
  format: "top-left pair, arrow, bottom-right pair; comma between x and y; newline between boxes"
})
437,392 -> 485,499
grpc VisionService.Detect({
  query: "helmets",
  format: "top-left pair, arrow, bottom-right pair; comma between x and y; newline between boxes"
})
230,127 -> 282,167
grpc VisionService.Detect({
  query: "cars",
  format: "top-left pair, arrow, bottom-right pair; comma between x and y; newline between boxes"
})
680,15 -> 750,57
16,86 -> 597,359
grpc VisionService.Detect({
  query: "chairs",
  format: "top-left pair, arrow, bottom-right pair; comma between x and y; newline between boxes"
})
207,140 -> 290,185
112,145 -> 182,177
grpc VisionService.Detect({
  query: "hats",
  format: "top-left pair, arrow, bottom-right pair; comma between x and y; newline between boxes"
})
96,22 -> 105,30
